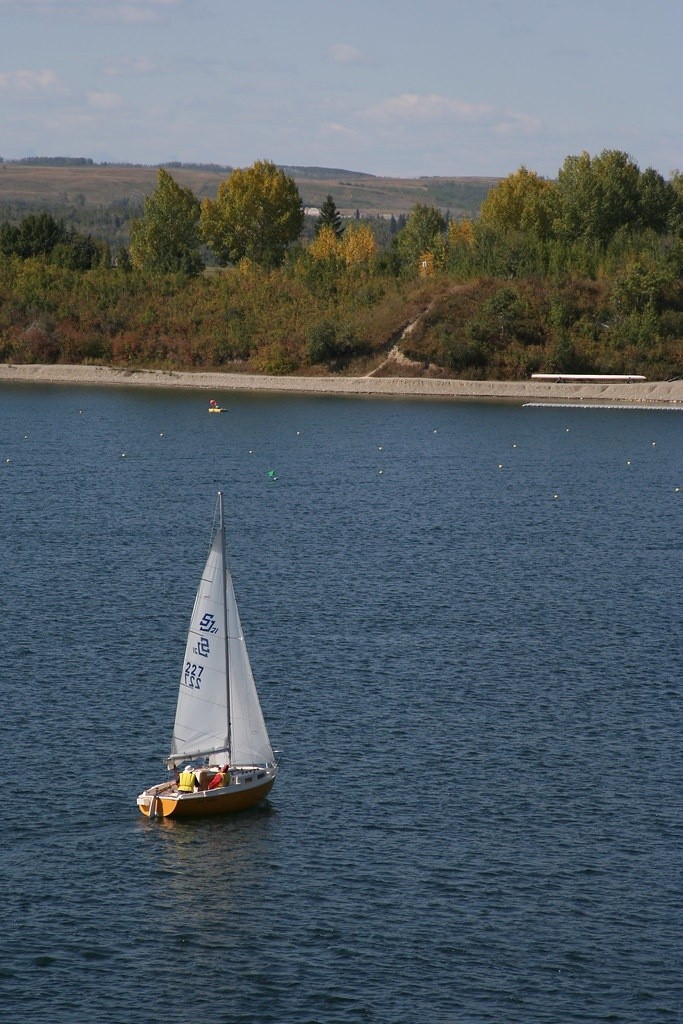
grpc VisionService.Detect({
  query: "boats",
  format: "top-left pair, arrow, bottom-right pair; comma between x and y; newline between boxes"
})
208,405 -> 227,413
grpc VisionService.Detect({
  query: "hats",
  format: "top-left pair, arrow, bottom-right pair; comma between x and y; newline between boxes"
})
184,765 -> 194,773
219,764 -> 225,768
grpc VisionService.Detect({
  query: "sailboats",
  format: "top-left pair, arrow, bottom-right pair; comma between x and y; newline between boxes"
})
137,491 -> 283,818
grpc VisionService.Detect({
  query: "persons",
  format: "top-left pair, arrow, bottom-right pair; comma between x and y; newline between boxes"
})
208,764 -> 230,789
176,765 -> 200,794
